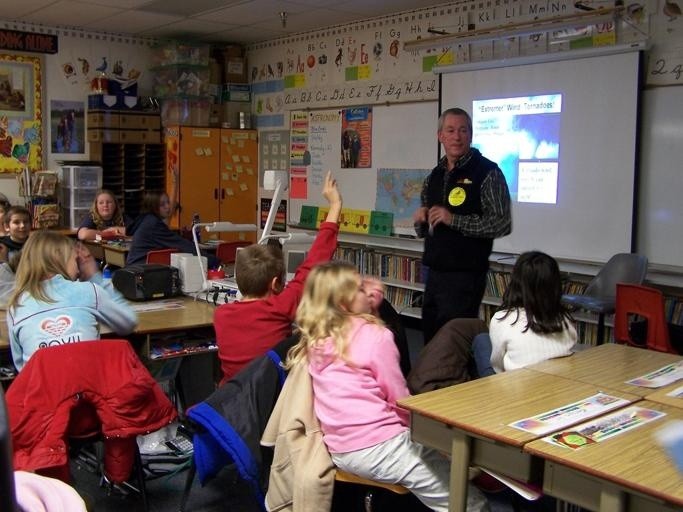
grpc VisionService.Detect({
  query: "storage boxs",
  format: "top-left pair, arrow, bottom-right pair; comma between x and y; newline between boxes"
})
87,110 -> 161,144
223,45 -> 252,128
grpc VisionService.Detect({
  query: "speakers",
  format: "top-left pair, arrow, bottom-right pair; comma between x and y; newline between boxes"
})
113,264 -> 182,302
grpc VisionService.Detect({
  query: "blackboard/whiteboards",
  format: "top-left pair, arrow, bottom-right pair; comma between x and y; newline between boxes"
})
287,99 -> 438,237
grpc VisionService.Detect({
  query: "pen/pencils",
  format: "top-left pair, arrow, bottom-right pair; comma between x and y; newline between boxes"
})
497,256 -> 514,260
211,264 -> 226,273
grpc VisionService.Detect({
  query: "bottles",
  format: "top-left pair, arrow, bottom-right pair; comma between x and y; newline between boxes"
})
193,215 -> 200,242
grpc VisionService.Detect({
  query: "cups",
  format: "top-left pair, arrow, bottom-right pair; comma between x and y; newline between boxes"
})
208,270 -> 225,279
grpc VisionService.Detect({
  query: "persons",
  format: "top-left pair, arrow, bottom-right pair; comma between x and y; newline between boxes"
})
0,197 -> 31,312
210,170 -> 343,390
125,189 -> 194,265
5,230 -> 139,376
469,250 -> 578,379
278,261 -> 491,512
412,108 -> 512,347
74,189 -> 127,241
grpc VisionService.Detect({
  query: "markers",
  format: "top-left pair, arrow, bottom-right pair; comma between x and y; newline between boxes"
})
399,234 -> 415,239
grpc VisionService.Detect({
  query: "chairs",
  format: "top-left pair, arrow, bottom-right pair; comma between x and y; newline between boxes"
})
10,340 -> 149,511
562,252 -> 648,345
614,283 -> 678,353
334,469 -> 408,512
146,248 -> 179,266
175,350 -> 224,511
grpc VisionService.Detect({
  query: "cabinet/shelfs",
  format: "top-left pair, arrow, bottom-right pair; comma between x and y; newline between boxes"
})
165,125 -> 257,244
334,241 -> 683,352
61,166 -> 103,230
101,143 -> 167,214
149,40 -> 212,100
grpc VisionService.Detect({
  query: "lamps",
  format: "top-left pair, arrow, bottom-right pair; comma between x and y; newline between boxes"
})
187,217 -> 256,292
254,168 -> 319,247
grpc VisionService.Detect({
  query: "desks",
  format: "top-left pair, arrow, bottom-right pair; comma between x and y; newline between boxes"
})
87,239 -> 133,268
0,297 -> 217,382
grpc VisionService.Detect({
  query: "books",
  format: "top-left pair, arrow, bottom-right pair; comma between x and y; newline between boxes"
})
333,247 -> 421,284
560,280 -> 683,326
574,320 -> 615,347
385,285 -> 414,307
484,270 -> 511,299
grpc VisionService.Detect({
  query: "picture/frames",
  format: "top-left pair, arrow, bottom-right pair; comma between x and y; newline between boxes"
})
48,97 -> 90,160
0,66 -> 31,117
